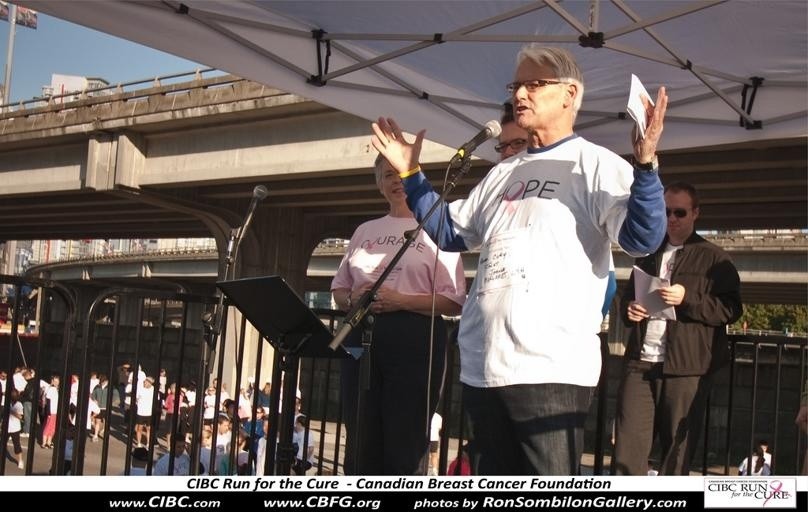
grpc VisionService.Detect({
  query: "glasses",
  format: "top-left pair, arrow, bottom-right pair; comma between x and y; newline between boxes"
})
666,207 -> 688,218
505,79 -> 568,94
494,138 -> 529,153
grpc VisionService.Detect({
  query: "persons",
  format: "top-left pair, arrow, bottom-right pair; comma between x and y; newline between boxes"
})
2,360 -> 315,479
793,379 -> 808,476
493,97 -> 621,394
738,445 -> 771,475
612,180 -> 744,476
368,45 -> 669,473
756,439 -> 772,475
327,153 -> 469,475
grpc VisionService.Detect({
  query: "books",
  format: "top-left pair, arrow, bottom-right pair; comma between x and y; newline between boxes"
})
631,264 -> 677,321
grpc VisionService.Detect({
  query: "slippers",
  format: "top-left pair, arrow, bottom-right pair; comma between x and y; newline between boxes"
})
46,443 -> 53,449
41,445 -> 48,449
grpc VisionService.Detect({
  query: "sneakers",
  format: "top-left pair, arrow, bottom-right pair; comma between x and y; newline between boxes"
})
91,435 -> 98,440
18,461 -> 24,469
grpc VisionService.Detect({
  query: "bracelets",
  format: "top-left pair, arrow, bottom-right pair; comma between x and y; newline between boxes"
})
344,293 -> 354,309
397,165 -> 421,179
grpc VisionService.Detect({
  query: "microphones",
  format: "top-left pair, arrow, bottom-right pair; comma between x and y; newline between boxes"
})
235,183 -> 269,247
448,119 -> 503,166
